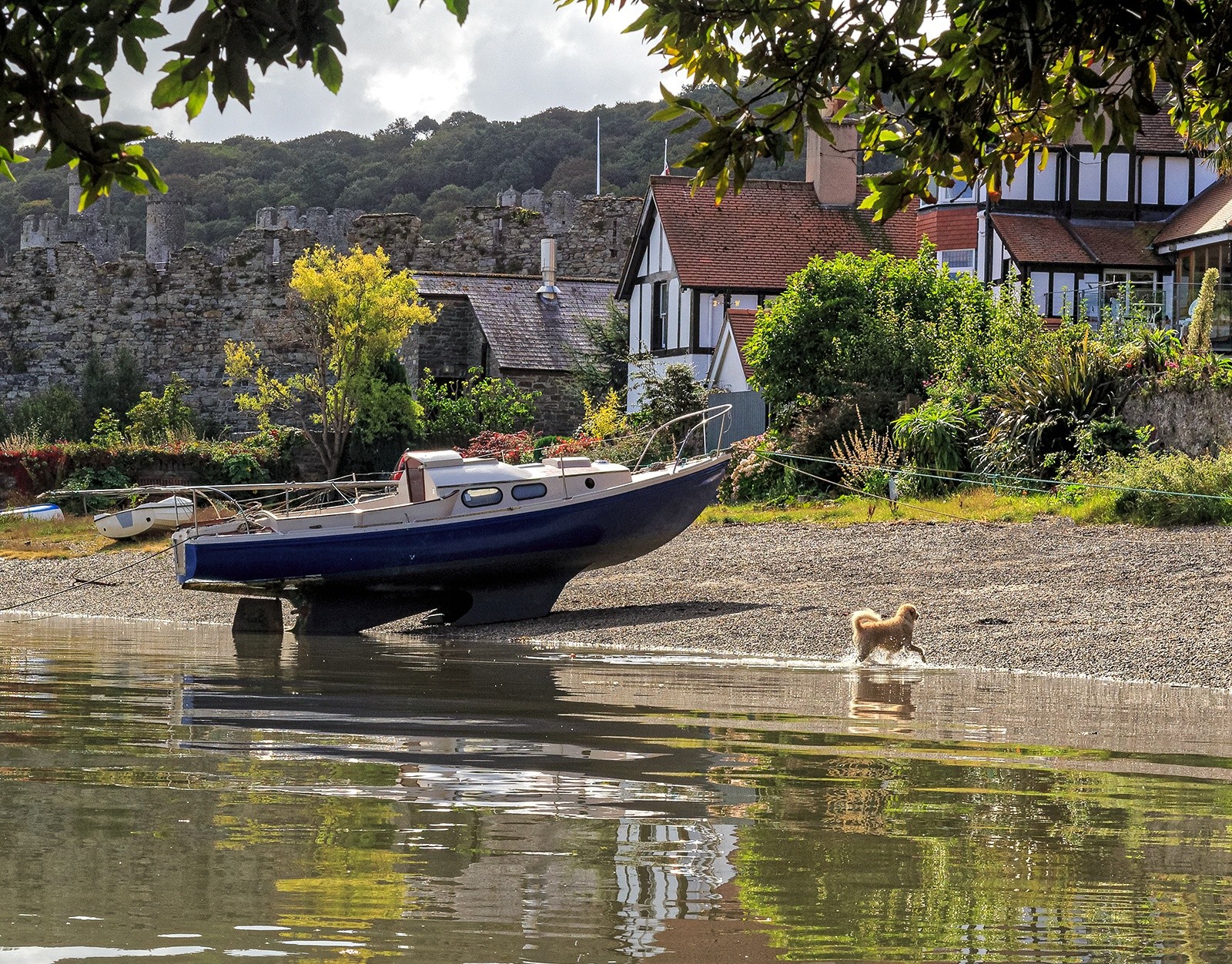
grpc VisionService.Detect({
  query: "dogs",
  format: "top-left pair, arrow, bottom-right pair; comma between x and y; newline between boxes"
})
852,605 -> 928,666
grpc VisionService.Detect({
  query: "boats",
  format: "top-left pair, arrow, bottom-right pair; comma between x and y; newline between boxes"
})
0,503 -> 66,523
92,493 -> 194,542
170,400 -> 740,636
